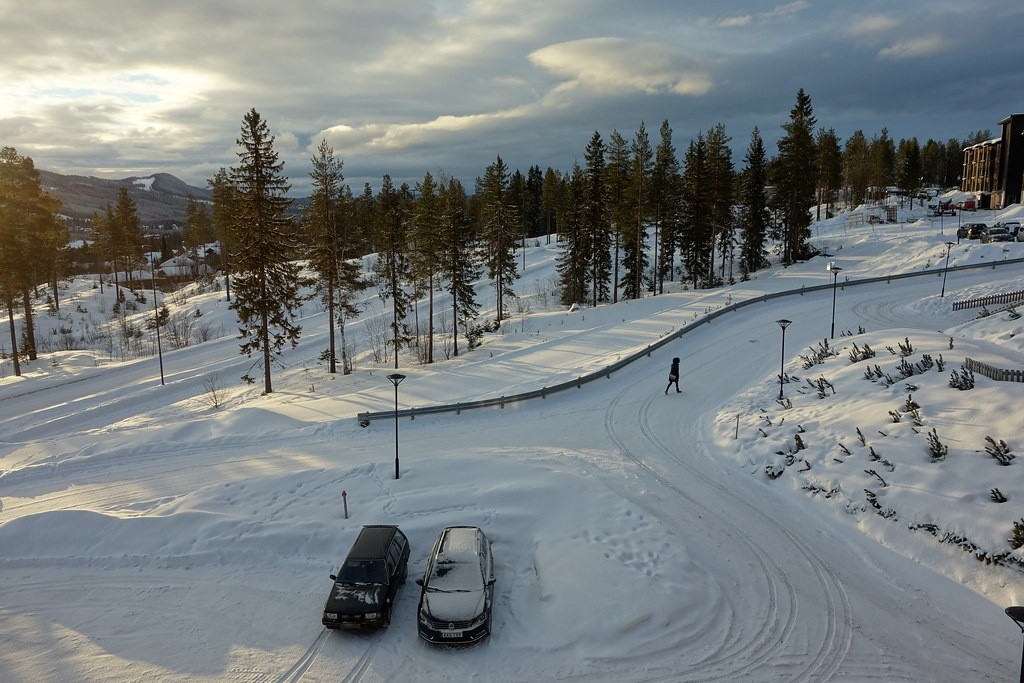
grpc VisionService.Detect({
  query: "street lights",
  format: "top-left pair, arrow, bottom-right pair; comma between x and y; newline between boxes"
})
941,241 -> 956,297
142,244 -> 164,385
385,373 -> 407,479
775,319 -> 793,400
830,266 -> 843,340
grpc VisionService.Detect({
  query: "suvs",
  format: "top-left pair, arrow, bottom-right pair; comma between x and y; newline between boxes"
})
415,525 -> 498,648
321,524 -> 411,632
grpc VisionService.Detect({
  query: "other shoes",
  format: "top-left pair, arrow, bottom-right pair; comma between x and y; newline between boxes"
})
664,390 -> 668,395
677,390 -> 682,393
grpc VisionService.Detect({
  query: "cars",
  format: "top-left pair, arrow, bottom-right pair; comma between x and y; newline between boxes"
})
1004,222 -> 1020,237
980,227 -> 1016,245
1017,225 -> 1024,241
957,223 -> 988,240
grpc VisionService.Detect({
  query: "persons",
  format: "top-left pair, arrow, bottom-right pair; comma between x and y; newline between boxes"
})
664,357 -> 682,395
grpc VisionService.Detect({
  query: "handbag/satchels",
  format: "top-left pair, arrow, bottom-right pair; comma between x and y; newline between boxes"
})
669,373 -> 676,381
